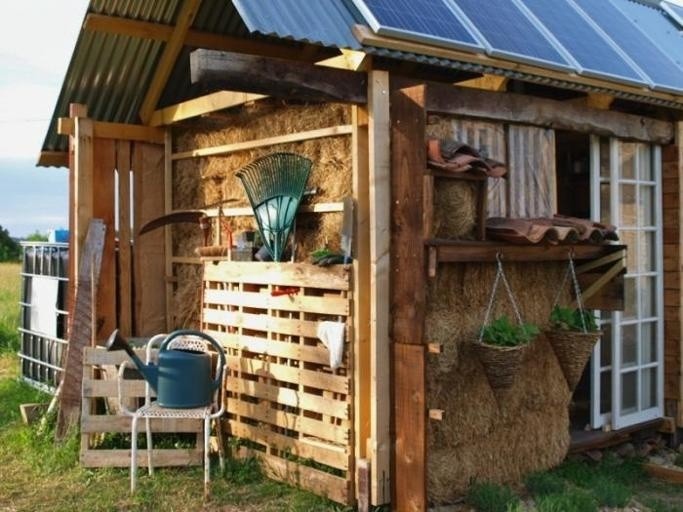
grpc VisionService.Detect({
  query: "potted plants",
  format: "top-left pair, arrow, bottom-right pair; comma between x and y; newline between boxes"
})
470,313 -> 540,418
542,306 -> 604,392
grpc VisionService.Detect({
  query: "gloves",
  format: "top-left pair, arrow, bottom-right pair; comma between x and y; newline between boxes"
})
308,250 -> 350,267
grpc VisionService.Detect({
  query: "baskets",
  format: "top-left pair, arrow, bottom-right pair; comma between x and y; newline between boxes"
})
474,340 -> 529,420
544,329 -> 602,395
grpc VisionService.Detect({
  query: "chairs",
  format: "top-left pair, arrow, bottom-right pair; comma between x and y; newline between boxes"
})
117,334 -> 226,502
